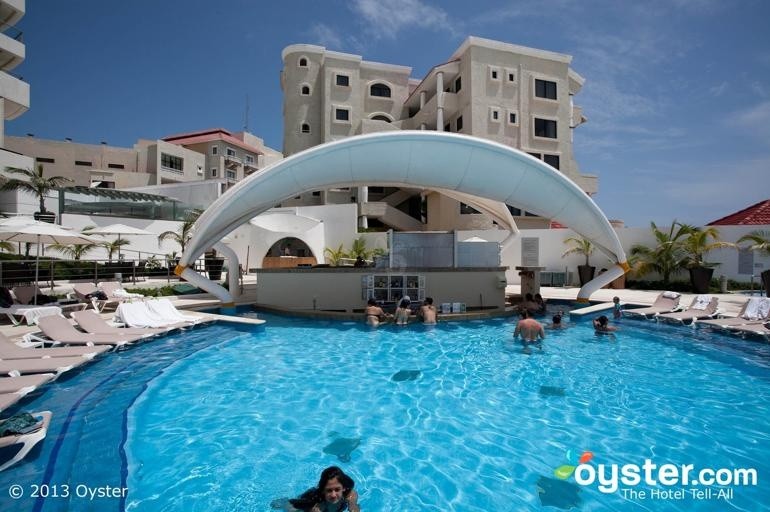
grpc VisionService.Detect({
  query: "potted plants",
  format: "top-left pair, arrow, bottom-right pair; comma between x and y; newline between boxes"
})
2,159 -> 75,223
352,235 -> 370,267
157,207 -> 206,281
561,226 -> 598,287
668,227 -> 770,297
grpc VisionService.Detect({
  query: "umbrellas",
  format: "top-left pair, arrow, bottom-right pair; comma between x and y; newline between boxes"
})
80,223 -> 158,261
0,215 -> 103,305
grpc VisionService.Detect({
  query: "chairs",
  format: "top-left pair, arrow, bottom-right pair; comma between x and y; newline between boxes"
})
104,261 -> 146,281
1,281 -> 197,471
620,286 -> 769,338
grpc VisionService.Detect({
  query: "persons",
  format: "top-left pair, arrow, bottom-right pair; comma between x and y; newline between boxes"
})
544,315 -> 567,329
418,297 -> 438,324
513,308 -> 546,342
611,296 -> 622,320
535,293 -> 546,315
392,299 -> 412,325
592,314 -> 620,331
557,309 -> 564,316
364,299 -> 388,326
354,255 -> 367,267
270,465 -> 363,512
516,294 -> 539,313
